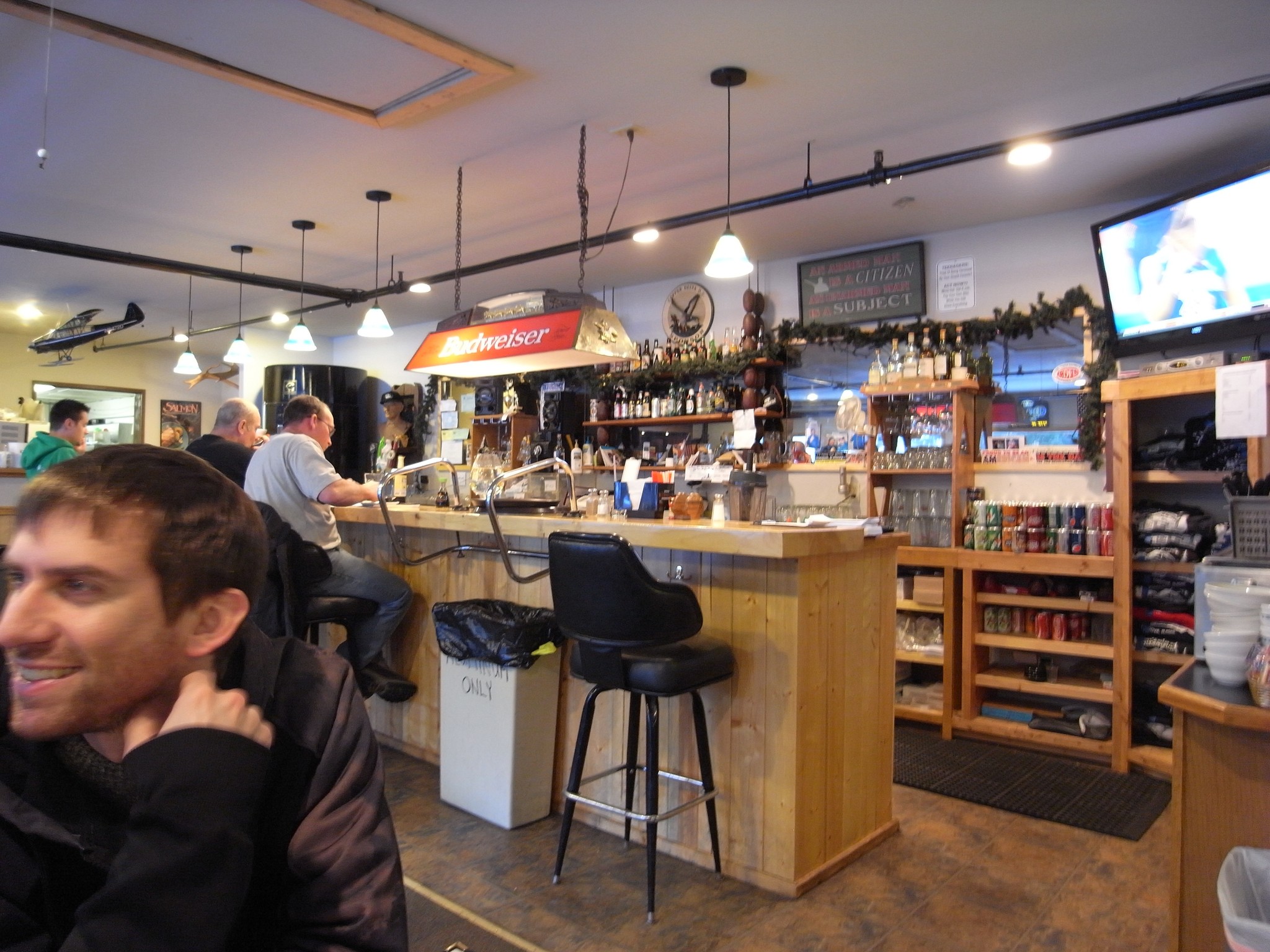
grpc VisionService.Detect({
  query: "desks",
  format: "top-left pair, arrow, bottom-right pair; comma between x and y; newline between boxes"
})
1155,658 -> 1270,952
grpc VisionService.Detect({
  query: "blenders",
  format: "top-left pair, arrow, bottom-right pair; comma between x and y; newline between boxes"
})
728,449 -> 768,521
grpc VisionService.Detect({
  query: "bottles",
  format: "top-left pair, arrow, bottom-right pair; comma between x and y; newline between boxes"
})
869,322 -> 992,386
555,433 -> 594,474
609,327 -> 771,420
435,477 -> 450,512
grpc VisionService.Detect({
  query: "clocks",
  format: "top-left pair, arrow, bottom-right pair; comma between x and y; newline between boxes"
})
663,282 -> 715,346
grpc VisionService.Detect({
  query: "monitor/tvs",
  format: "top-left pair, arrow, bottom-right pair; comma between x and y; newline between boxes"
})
1089,161 -> 1270,357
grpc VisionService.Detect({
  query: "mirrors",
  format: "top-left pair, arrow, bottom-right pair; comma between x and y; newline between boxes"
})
766,304 -> 1094,477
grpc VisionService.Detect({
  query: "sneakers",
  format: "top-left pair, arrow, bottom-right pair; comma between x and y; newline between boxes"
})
331,640 -> 375,700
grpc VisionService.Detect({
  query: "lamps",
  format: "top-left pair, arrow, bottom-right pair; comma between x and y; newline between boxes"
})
173,271 -> 203,376
224,242 -> 254,366
282,218 -> 319,354
355,189 -> 399,341
700,64 -> 755,282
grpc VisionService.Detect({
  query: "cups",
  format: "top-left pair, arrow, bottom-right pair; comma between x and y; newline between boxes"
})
471,452 -> 504,498
873,444 -> 952,549
777,504 -> 861,524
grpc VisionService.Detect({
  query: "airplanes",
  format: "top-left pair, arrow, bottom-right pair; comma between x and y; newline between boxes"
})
26,300 -> 145,369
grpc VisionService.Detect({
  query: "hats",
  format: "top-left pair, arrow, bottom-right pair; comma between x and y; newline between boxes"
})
380,390 -> 404,405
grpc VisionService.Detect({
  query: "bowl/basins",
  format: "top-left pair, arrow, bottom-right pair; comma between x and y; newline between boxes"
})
1203,582 -> 1270,687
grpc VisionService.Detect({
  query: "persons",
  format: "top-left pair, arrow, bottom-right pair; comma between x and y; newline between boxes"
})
376,392 -> 425,467
19,399 -> 91,480
245,394 -> 418,702
188,399 -> 265,488
0,445 -> 410,952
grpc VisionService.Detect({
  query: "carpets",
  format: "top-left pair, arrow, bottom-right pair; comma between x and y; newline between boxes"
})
892,730 -> 1174,842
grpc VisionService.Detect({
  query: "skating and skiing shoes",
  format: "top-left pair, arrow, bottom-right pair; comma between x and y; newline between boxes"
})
360,662 -> 418,703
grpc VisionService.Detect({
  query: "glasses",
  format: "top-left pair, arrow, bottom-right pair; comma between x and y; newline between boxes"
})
307,415 -> 336,437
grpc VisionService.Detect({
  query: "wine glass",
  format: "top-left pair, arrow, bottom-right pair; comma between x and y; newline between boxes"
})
880,391 -> 954,435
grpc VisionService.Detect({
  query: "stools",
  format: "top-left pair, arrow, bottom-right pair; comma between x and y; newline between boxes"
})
543,528 -> 736,923
254,503 -> 382,687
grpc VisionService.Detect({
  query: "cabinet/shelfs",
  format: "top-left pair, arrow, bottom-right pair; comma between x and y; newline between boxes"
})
574,363 -> 787,482
858,353 -> 1270,783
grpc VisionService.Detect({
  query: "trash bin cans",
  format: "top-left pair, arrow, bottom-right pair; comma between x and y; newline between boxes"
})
433,599 -> 562,831
1215,847 -> 1269,952
